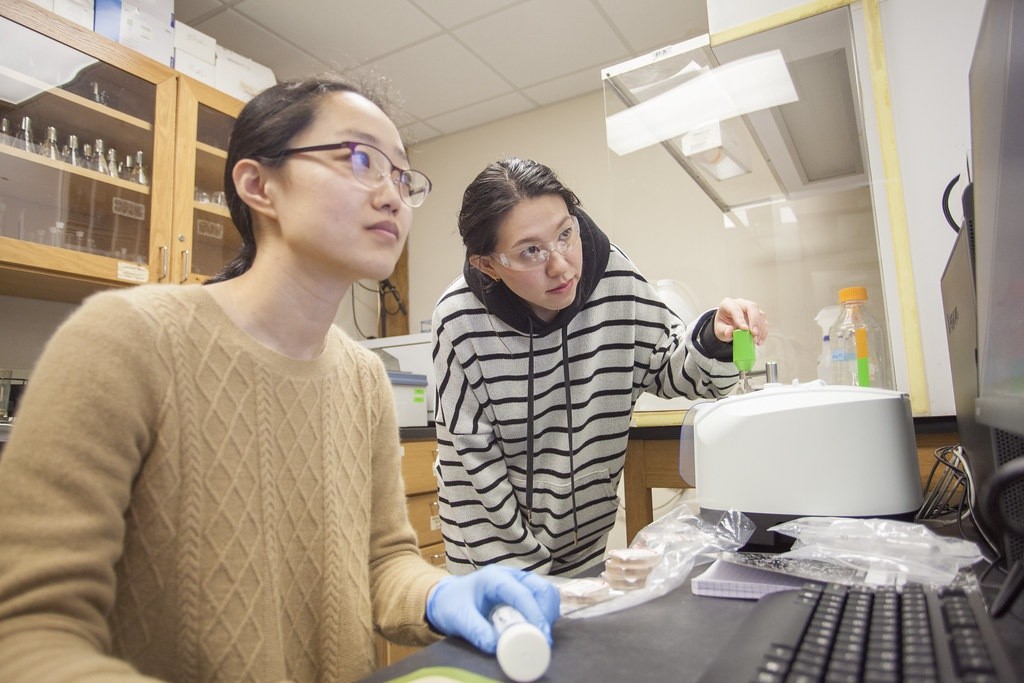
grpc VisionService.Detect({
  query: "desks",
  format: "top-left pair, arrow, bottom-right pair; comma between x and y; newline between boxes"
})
353,508 -> 1022,682
623,410 -> 962,550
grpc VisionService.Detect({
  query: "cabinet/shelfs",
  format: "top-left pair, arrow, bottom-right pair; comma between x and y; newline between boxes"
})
0,0 -> 254,303
395,427 -> 446,573
599,0 -> 868,214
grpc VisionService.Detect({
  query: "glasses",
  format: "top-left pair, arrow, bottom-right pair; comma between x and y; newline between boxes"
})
488,214 -> 580,273
285,141 -> 433,210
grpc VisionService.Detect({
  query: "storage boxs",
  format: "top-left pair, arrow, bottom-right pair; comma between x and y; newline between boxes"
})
27,0 -> 278,106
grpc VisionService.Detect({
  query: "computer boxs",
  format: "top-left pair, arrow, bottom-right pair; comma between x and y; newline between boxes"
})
940,215 -> 1023,575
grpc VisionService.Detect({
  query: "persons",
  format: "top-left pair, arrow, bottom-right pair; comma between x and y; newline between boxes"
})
430,156 -> 769,580
0,73 -> 569,682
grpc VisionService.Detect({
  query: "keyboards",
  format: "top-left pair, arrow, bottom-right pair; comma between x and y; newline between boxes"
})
699,581 -> 1016,683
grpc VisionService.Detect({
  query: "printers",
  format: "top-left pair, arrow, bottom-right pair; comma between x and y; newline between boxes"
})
679,382 -> 925,547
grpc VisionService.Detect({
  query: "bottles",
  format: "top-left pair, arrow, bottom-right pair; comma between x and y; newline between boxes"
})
1,115 -> 150,262
827,286 -> 889,388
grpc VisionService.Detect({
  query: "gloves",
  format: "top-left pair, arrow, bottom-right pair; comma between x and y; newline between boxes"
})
425,563 -> 561,655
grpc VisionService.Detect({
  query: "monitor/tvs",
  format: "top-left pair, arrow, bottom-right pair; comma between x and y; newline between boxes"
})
966,0 -> 1024,439
